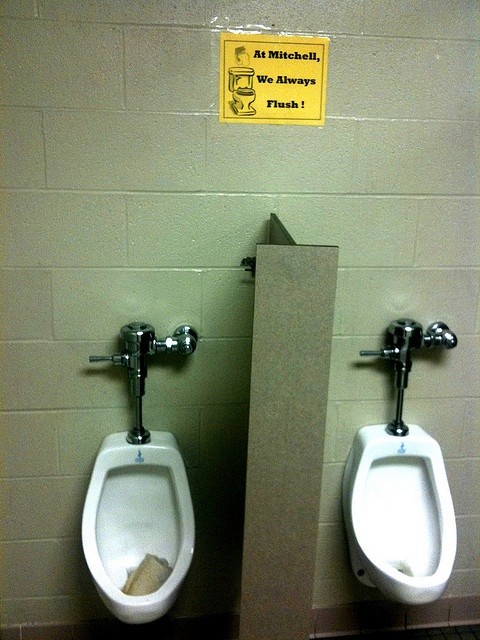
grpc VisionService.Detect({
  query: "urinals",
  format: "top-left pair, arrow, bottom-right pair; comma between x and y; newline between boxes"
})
341,314 -> 460,608
80,322 -> 200,627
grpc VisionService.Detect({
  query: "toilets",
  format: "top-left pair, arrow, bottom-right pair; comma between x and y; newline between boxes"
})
228,66 -> 257,116
